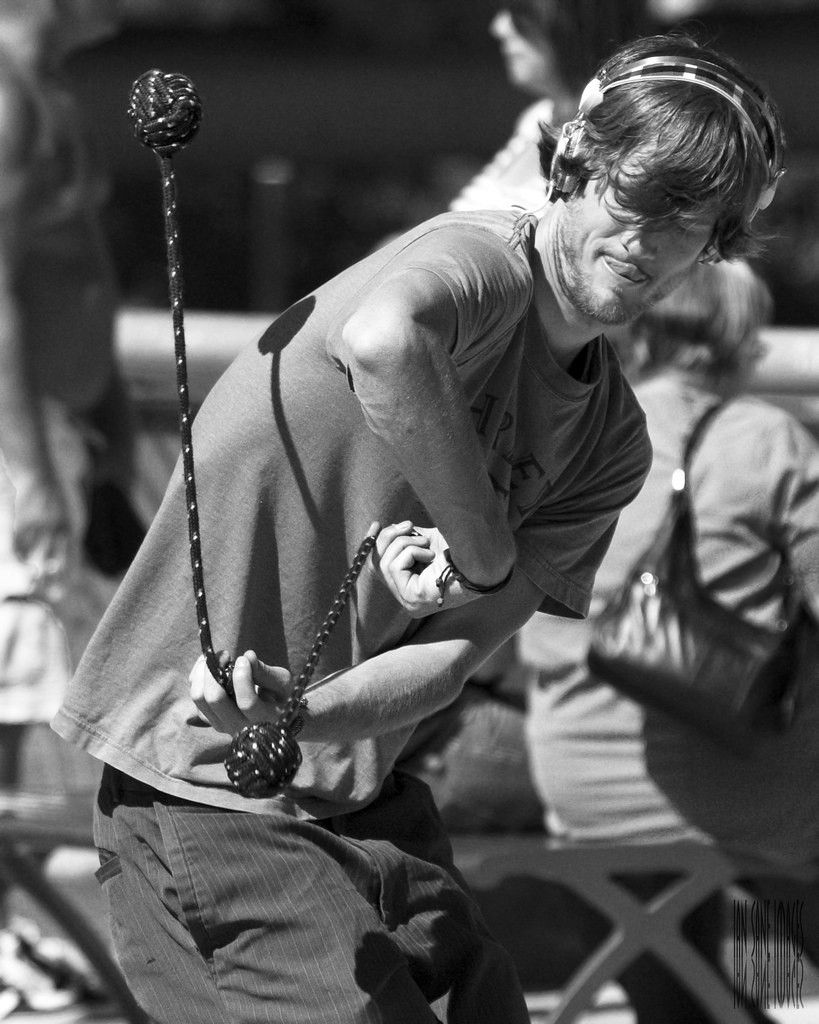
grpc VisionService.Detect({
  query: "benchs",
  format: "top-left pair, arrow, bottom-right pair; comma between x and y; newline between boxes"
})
0,790 -> 761,1024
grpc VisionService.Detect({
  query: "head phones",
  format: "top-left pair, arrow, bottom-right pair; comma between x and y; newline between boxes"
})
547,56 -> 789,268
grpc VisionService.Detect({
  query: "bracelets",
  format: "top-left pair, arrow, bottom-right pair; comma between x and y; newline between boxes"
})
436,548 -> 515,608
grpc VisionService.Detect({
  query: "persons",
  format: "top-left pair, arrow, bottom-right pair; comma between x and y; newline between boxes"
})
50,34 -> 786,1024
518,251 -> 818,868
448,1 -> 650,211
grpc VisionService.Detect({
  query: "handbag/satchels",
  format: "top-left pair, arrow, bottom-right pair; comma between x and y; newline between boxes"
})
586,390 -> 807,750
